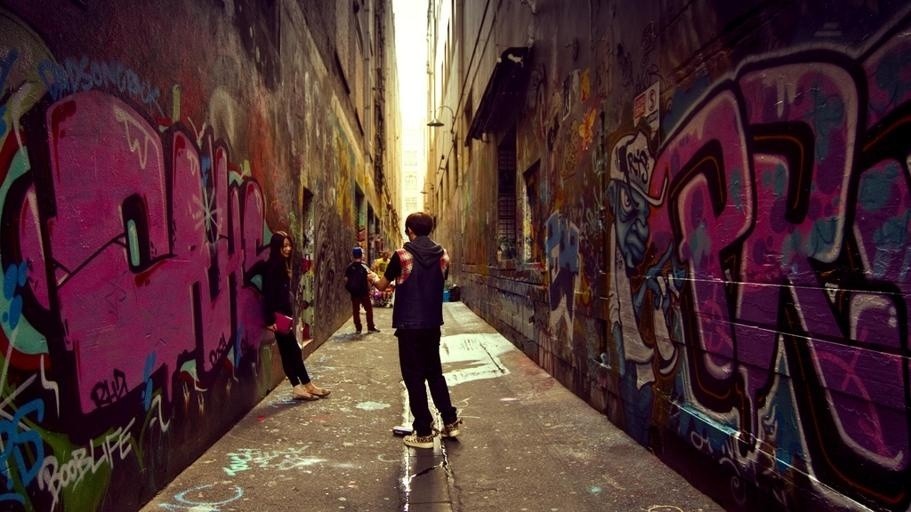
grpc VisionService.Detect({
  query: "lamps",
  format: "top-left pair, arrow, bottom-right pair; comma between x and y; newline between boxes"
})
427,105 -> 457,140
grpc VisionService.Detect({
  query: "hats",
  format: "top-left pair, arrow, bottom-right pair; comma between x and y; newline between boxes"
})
352,247 -> 362,253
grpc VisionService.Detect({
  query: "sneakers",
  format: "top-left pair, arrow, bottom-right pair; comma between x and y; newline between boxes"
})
356,326 -> 381,334
403,430 -> 434,449
293,388 -> 331,401
440,424 -> 461,438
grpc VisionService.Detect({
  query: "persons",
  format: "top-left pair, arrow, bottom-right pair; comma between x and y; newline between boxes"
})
366,212 -> 461,449
343,247 -> 381,334
261,230 -> 332,402
370,251 -> 396,308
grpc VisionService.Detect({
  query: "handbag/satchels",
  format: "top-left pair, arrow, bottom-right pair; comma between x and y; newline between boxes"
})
275,312 -> 295,335
